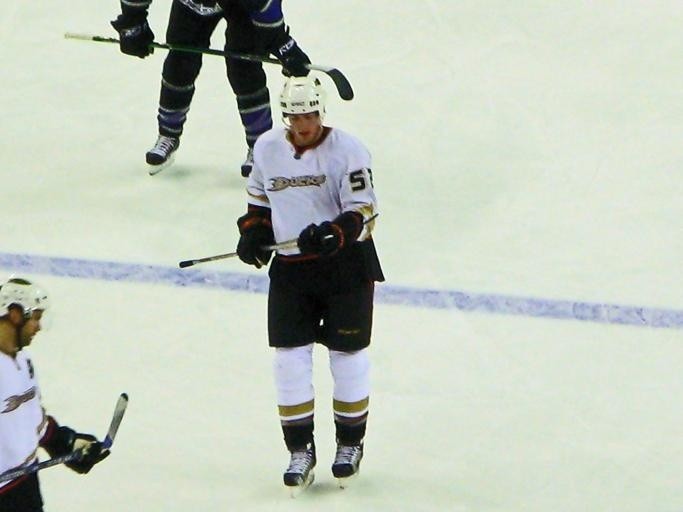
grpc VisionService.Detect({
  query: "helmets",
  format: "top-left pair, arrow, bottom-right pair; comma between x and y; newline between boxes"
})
279,74 -> 326,122
0,275 -> 49,318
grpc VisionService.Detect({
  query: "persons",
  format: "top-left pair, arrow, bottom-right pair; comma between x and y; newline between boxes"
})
235,75 -> 385,487
111,0 -> 312,177
0,279 -> 110,512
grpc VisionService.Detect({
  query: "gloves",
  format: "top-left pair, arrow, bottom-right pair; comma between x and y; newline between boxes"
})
236,210 -> 275,268
296,220 -> 341,256
268,33 -> 312,77
50,425 -> 112,474
109,11 -> 155,59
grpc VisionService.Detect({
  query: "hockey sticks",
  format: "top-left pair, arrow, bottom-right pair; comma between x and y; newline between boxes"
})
64,32 -> 354,101
0,393 -> 129,481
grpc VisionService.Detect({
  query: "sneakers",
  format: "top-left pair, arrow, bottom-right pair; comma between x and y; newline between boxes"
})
283,443 -> 316,487
145,135 -> 181,165
331,439 -> 364,478
